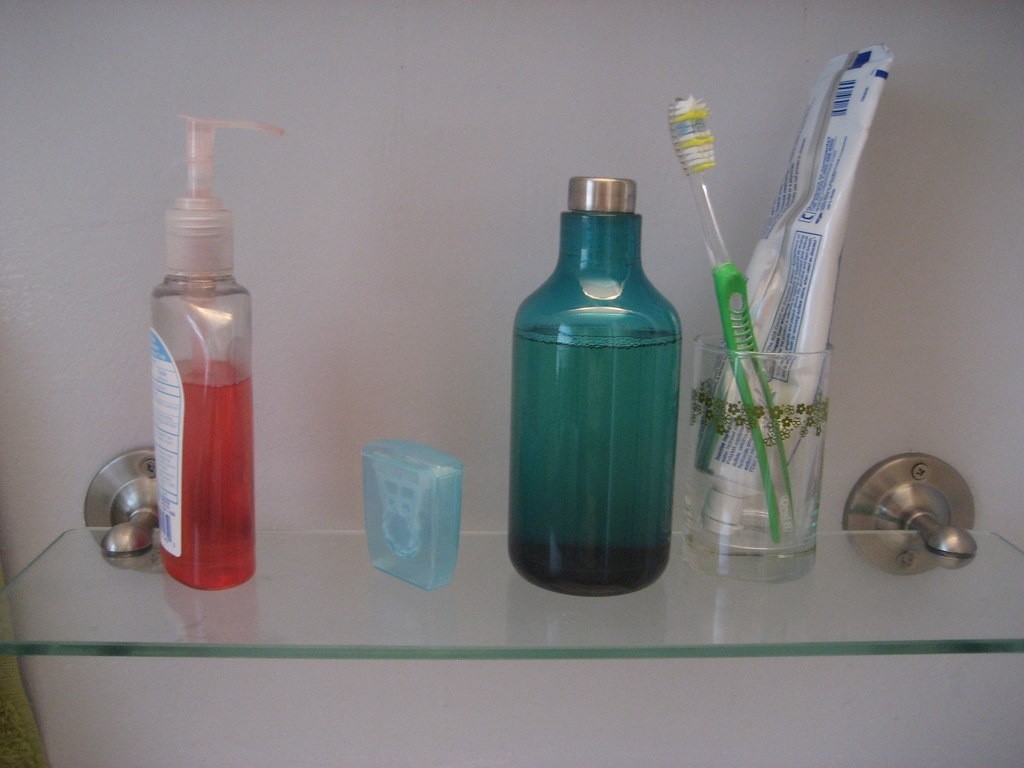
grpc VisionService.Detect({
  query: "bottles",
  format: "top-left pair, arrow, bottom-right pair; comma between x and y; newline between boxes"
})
506,173 -> 685,597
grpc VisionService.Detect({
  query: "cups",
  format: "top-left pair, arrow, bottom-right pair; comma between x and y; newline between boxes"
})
674,332 -> 836,587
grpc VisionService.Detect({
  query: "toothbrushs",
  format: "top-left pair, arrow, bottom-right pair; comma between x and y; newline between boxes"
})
666,92 -> 796,547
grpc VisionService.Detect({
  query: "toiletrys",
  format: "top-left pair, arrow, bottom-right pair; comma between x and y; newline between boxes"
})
698,42 -> 893,536
150,111 -> 286,589
506,175 -> 682,598
